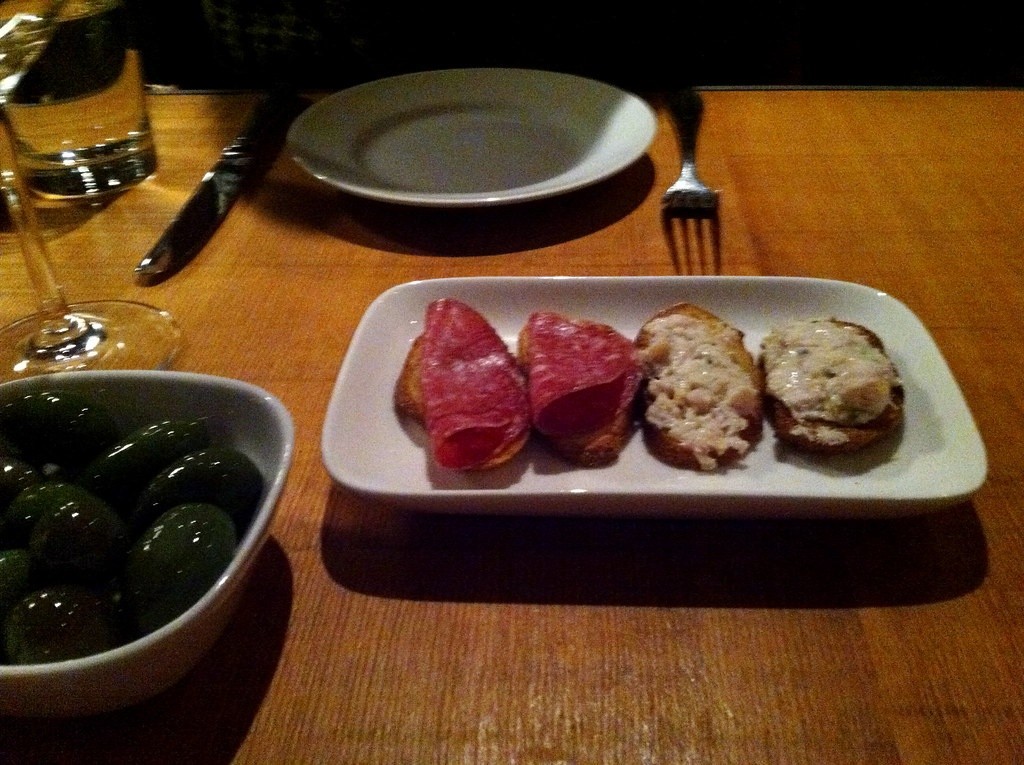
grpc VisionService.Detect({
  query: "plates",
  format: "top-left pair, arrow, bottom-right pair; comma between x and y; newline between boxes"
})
319,276 -> 989,518
287,68 -> 656,205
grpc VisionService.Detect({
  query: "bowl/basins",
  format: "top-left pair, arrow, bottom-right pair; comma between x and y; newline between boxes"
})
0,370 -> 295,721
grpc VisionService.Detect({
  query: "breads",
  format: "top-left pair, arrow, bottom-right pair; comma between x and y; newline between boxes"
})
517,310 -> 642,467
636,301 -> 764,470
757,320 -> 903,452
394,333 -> 531,472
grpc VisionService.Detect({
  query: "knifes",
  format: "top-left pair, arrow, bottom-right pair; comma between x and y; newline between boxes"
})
135,93 -> 313,277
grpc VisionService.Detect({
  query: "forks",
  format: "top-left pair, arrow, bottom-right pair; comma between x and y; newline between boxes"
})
660,84 -> 720,214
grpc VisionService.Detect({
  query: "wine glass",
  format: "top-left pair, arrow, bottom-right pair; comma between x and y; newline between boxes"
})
0,0 -> 182,377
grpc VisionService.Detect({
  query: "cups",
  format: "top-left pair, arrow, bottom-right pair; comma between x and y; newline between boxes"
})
4,1 -> 158,202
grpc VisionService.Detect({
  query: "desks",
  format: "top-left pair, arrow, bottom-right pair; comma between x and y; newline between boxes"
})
0,84 -> 1024,765
